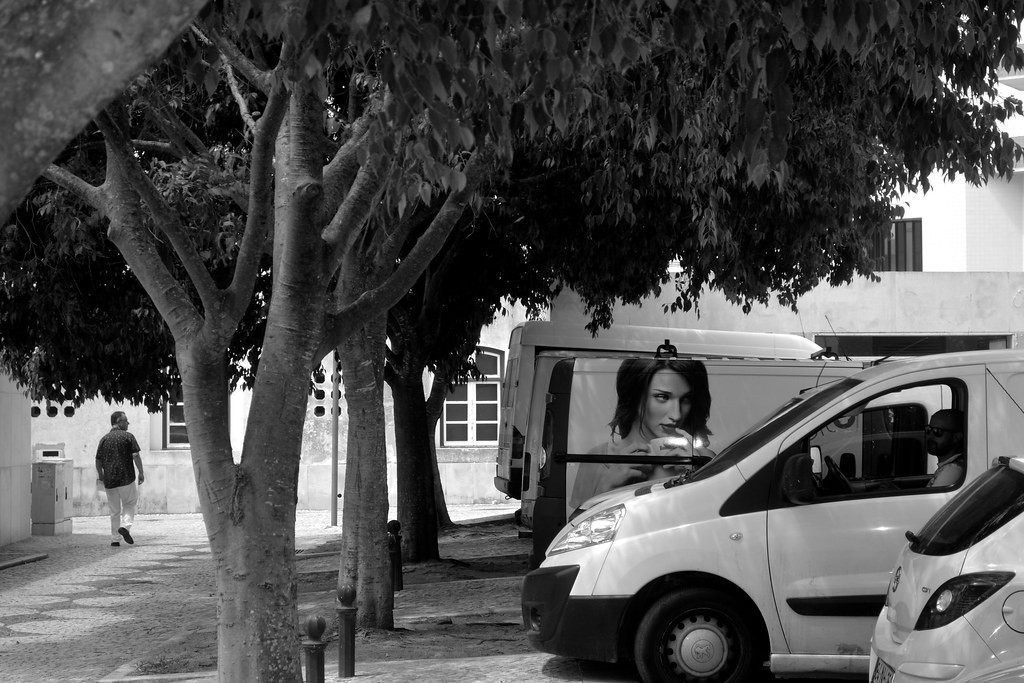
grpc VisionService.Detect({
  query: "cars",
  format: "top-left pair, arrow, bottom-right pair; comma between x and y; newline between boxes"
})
869,456 -> 1024,683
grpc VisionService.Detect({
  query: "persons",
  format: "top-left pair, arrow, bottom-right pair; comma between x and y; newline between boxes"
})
94,411 -> 145,545
568,358 -> 718,508
922,409 -> 964,488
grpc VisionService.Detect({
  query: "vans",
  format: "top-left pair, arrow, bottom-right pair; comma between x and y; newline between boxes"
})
494,323 -> 836,501
520,340 -> 893,571
522,349 -> 1024,683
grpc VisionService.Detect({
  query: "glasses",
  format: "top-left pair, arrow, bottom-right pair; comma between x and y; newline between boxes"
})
925,425 -> 956,438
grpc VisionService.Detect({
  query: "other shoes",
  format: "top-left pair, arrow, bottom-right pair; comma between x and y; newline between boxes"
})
111,542 -> 120,546
118,527 -> 134,544
